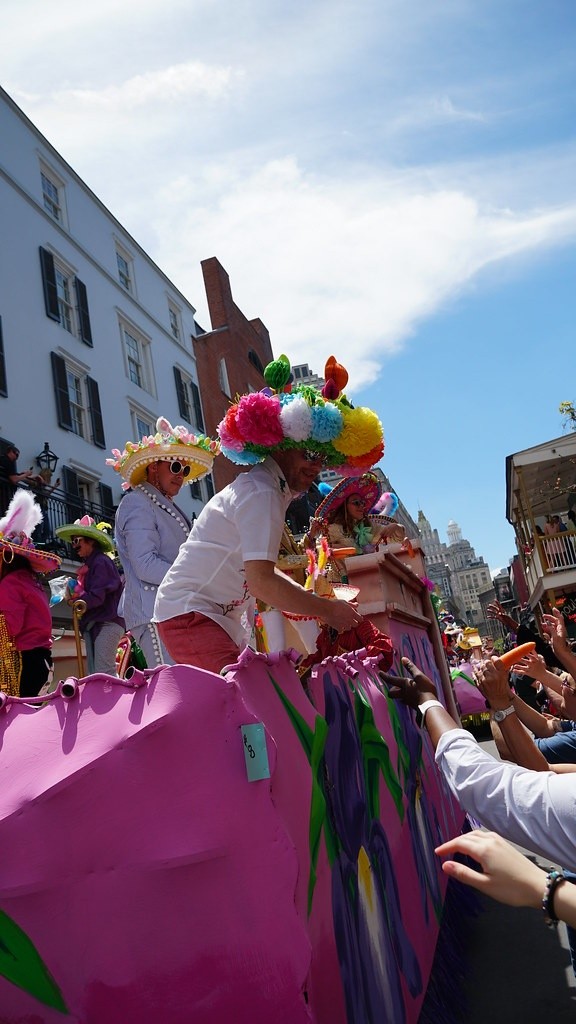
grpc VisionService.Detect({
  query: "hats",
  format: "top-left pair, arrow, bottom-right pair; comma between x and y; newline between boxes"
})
215,354 -> 386,479
106,415 -> 221,491
55,515 -> 114,553
313,472 -> 381,526
0,488 -> 62,573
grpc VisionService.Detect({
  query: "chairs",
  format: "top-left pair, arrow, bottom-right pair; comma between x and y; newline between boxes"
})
41,531 -> 68,559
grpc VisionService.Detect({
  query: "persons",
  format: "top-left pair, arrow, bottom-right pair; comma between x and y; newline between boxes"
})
530,510 -> 576,573
151,447 -> 384,674
54,515 -> 125,678
302,470 -> 405,585
377,598 -> 576,976
28,469 -> 60,539
0,530 -> 62,710
106,417 -> 221,669
0,445 -> 33,518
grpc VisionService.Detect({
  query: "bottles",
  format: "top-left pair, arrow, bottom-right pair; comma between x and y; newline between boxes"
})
340,575 -> 349,584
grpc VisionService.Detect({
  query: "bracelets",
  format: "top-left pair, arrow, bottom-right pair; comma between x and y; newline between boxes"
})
540,865 -> 565,929
304,532 -> 312,543
508,692 -> 515,701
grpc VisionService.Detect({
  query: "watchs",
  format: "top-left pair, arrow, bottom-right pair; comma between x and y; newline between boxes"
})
493,705 -> 515,721
415,700 -> 444,731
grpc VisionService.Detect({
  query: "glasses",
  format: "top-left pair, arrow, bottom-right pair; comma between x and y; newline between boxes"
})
164,460 -> 191,477
305,450 -> 330,466
350,500 -> 366,507
13,451 -> 18,457
562,680 -> 576,693
72,537 -> 84,544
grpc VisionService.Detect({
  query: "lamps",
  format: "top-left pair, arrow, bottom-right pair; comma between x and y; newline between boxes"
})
36,442 -> 59,476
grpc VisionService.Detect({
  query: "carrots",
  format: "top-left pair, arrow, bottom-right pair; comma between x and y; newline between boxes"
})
500,642 -> 536,668
331,547 -> 356,559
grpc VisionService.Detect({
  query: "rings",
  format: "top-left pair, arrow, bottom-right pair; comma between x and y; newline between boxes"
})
338,631 -> 343,634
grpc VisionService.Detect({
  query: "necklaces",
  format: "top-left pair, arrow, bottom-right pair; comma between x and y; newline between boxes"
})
0,614 -> 22,697
138,484 -> 189,536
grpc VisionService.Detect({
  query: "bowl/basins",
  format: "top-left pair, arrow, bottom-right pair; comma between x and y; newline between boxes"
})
332,583 -> 360,601
